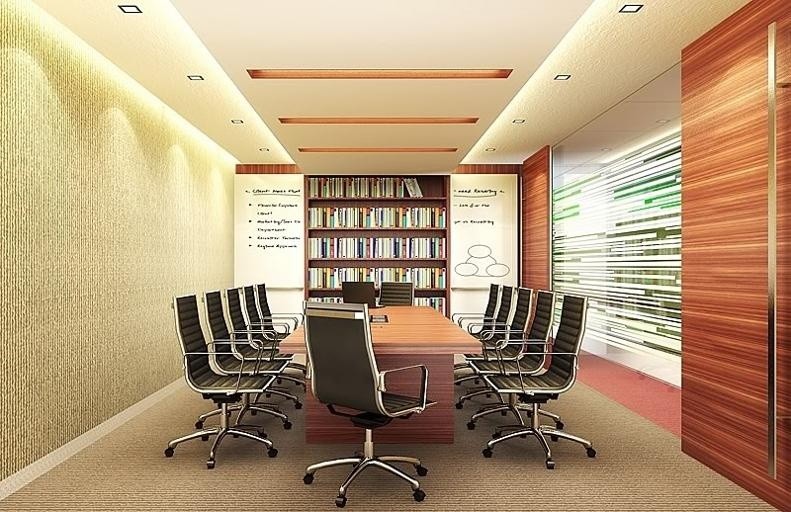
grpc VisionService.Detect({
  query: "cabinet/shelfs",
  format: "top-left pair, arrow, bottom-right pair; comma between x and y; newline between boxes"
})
303,174 -> 452,319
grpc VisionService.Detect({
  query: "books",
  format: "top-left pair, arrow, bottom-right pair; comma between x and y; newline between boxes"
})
370,314 -> 389,324
305,177 -> 446,318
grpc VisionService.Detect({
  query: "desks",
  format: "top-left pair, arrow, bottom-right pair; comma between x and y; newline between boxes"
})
303,306 -> 482,445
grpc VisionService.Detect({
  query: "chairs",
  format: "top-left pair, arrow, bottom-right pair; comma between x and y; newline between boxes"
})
256,285 -> 307,384
165,293 -> 278,469
302,300 -> 438,507
379,283 -> 416,306
455,287 -> 535,416
224,288 -> 303,416
194,289 -> 293,430
454,285 -> 513,398
482,295 -> 594,470
454,283 -> 500,385
242,285 -> 307,398
467,290 -> 565,438
341,282 -> 377,308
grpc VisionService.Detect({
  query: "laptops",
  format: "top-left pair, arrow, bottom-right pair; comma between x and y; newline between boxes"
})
342,281 -> 385,308
